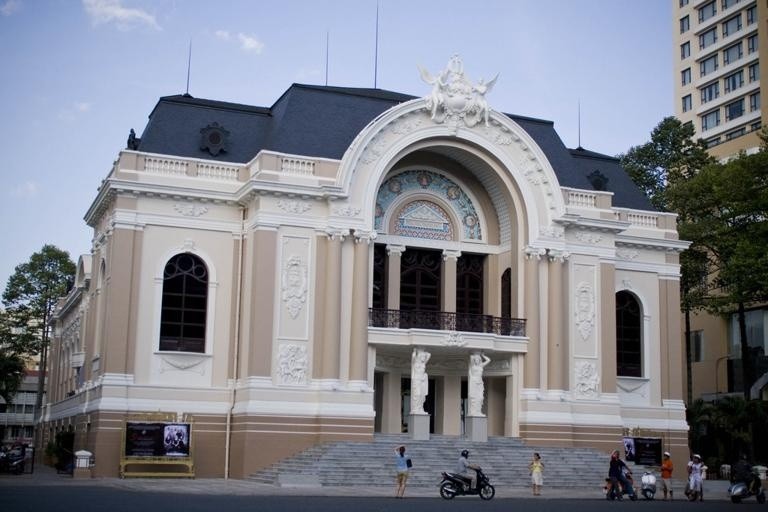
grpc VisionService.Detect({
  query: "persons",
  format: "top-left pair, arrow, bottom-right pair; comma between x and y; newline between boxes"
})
608,449 -> 636,499
686,453 -> 709,500
467,349 -> 492,419
527,454 -> 546,496
687,455 -> 705,501
659,450 -> 675,500
170,429 -> 185,454
393,444 -> 409,499
410,348 -> 432,416
456,448 -> 481,493
733,453 -> 757,494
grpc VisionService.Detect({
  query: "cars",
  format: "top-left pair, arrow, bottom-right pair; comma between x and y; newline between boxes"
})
1,442 -> 33,475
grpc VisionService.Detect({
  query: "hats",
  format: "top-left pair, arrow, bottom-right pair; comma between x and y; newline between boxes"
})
664,452 -> 670,457
693,454 -> 701,459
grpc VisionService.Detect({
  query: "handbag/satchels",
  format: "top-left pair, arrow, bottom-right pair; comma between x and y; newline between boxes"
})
406,458 -> 413,468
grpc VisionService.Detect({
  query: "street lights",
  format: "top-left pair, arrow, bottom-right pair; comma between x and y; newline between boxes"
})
715,353 -> 736,401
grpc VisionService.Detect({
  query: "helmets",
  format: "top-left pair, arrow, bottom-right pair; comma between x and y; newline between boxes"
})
461,450 -> 469,459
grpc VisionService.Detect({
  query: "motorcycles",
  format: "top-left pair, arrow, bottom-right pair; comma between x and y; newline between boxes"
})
439,465 -> 495,500
640,469 -> 658,499
728,471 -> 766,504
603,471 -> 638,501
